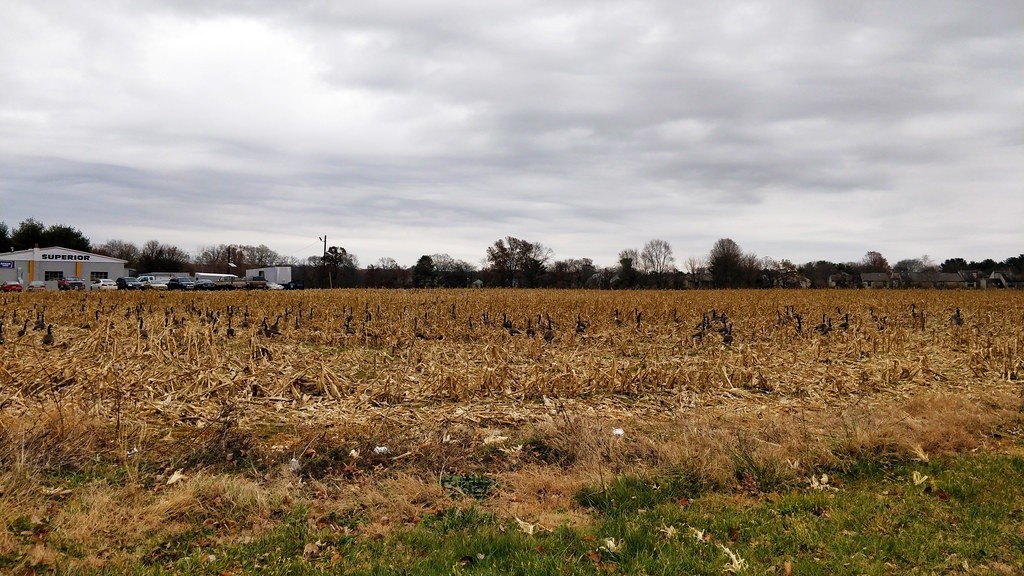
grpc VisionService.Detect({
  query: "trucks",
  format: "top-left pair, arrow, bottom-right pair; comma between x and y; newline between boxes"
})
136,276 -> 169,290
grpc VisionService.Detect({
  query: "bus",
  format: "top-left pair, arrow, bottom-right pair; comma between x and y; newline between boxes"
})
194,273 -> 238,286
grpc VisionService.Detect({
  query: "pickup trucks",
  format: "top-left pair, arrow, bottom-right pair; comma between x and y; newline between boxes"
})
231,276 -> 268,289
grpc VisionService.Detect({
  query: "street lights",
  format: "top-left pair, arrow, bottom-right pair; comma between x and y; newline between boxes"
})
319,235 -> 326,289
226,246 -> 230,273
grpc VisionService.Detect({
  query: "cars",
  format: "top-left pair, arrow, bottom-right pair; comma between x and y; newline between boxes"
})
90,279 -> 118,291
116,277 -> 145,291
0,280 -> 23,292
168,277 -> 197,291
772,276 -> 811,289
57,275 -> 86,290
144,281 -> 167,290
195,279 -> 216,291
25,281 -> 47,292
